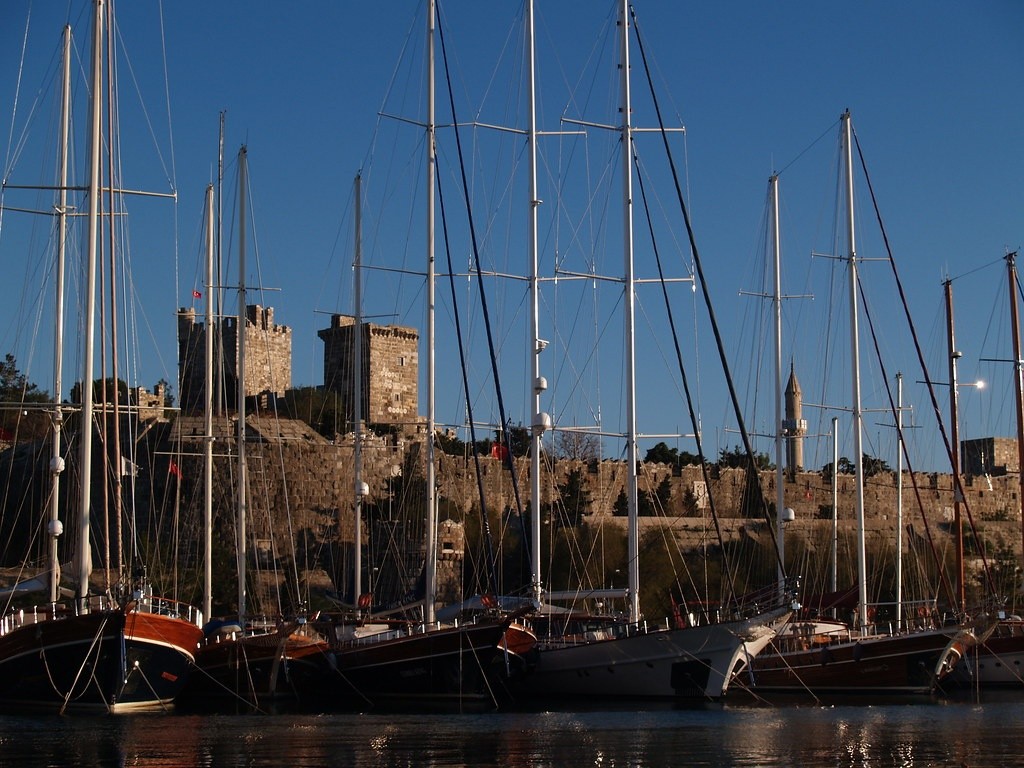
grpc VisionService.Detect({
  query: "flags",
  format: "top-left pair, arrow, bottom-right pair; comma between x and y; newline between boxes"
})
193,290 -> 201,299
121,456 -> 139,477
169,462 -> 183,479
491,441 -> 509,461
805,491 -> 812,501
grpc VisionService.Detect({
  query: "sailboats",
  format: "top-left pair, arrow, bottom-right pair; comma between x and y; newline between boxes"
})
0,0 -> 1024,722
